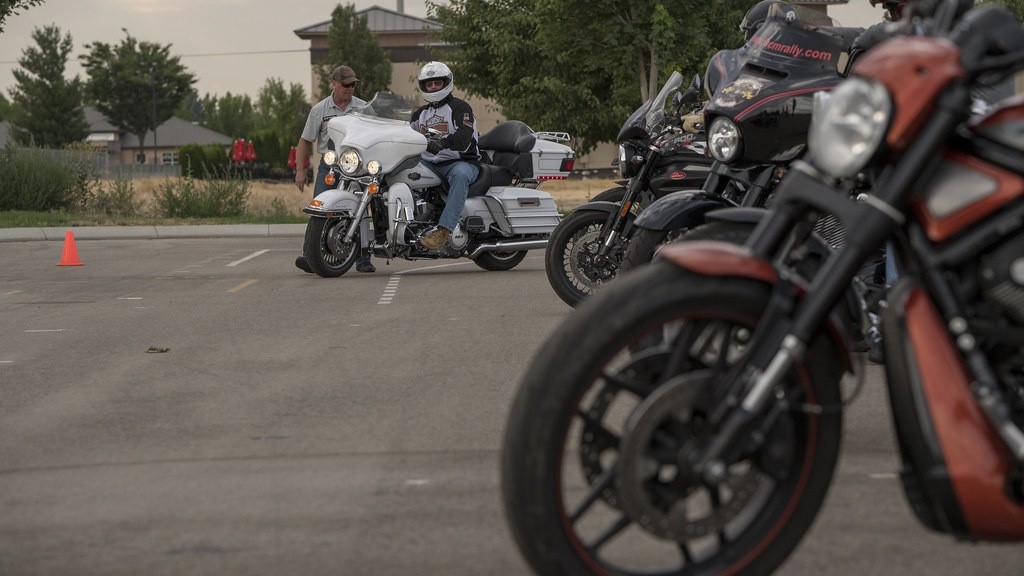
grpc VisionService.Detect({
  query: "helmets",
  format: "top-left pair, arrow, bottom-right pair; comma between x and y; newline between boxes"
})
740,0 -> 798,42
417,61 -> 454,103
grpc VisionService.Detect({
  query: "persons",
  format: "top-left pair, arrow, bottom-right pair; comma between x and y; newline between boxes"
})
293,65 -> 376,273
410,61 -> 481,248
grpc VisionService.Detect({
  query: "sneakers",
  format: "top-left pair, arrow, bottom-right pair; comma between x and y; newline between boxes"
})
420,228 -> 450,250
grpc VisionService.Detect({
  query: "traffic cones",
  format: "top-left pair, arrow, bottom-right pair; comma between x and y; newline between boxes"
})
56,230 -> 85,266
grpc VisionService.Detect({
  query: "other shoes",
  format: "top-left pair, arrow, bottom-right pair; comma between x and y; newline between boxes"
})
356,262 -> 377,273
295,256 -> 315,273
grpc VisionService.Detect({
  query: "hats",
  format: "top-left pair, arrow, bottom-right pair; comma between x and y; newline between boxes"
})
330,66 -> 359,84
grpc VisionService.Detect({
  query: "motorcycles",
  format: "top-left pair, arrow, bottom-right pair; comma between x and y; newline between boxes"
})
499,0 -> 1023,576
302,90 -> 564,278
584,100 -> 710,214
618,0 -> 865,382
544,71 -> 719,310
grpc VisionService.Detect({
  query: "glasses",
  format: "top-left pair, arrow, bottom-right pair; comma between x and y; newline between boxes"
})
425,81 -> 444,88
336,82 -> 357,88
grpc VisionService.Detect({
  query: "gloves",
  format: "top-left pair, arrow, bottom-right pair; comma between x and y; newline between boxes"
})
425,140 -> 445,155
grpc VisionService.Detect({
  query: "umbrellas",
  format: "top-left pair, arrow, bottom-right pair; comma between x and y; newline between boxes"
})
232,138 -> 256,179
288,146 -> 310,182
